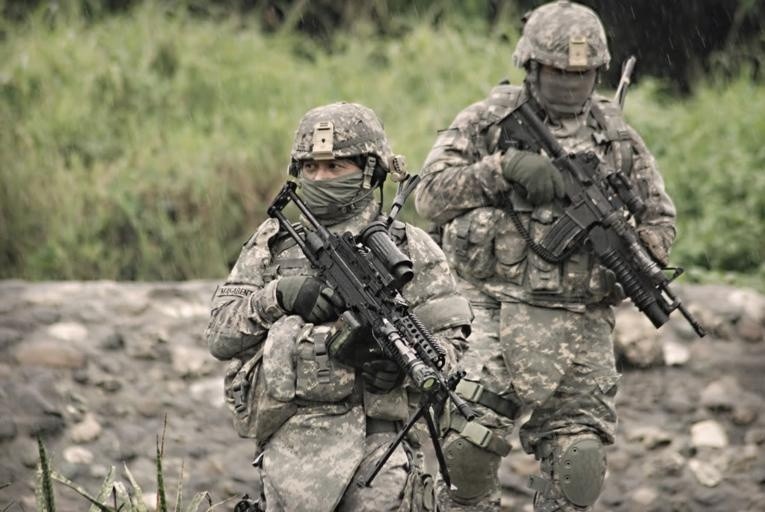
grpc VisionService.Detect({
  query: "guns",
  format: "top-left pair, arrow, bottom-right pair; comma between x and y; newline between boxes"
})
494,98 -> 707,339
266,181 -> 476,422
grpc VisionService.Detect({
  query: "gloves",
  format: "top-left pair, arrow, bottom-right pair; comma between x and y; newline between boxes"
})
500,145 -> 566,204
361,345 -> 407,397
277,275 -> 348,326
597,263 -> 626,303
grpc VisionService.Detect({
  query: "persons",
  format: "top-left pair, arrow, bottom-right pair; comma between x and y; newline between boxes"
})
415,0 -> 677,512
204,97 -> 471,512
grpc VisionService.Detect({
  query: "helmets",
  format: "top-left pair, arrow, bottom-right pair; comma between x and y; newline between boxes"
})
290,101 -> 395,175
512,0 -> 613,74
